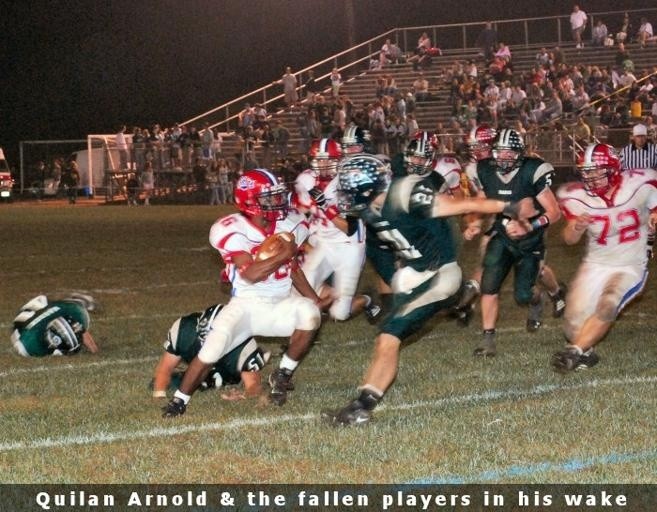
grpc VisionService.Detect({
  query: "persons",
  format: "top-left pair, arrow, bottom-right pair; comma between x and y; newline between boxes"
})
8,291 -> 100,358
66,152 -> 83,203
116,2 -> 657,429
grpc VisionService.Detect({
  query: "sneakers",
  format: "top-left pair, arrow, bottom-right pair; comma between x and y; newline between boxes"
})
449,279 -> 479,328
269,369 -> 291,408
160,398 -> 186,419
473,338 -> 498,359
364,291 -> 383,325
318,401 -> 371,427
549,281 -> 567,318
550,344 -> 600,371
526,292 -> 544,333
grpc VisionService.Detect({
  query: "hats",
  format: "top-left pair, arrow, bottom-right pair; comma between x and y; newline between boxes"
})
632,124 -> 647,137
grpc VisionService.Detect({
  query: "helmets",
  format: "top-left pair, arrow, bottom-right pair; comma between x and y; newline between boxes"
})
576,143 -> 622,198
195,303 -> 226,349
308,138 -> 342,181
232,167 -> 289,222
491,127 -> 523,174
403,141 -> 434,175
414,130 -> 439,148
339,153 -> 390,211
341,125 -> 375,154
466,126 -> 497,160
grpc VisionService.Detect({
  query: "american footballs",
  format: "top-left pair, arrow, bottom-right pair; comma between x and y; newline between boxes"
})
252,232 -> 294,261
